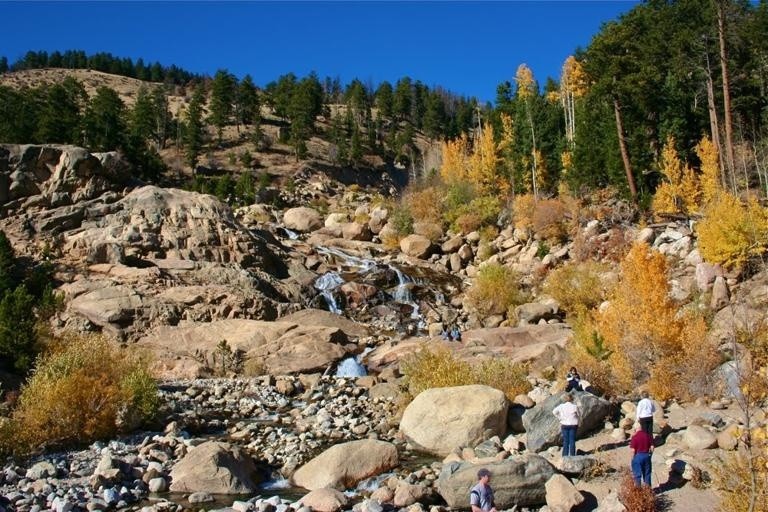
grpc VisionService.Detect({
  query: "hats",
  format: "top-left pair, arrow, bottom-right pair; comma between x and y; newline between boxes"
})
478,469 -> 493,476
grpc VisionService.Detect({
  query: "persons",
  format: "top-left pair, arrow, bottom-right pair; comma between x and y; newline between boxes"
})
636,390 -> 658,452
564,366 -> 584,392
441,324 -> 449,338
450,324 -> 461,341
469,467 -> 499,512
552,392 -> 581,457
629,420 -> 654,489
